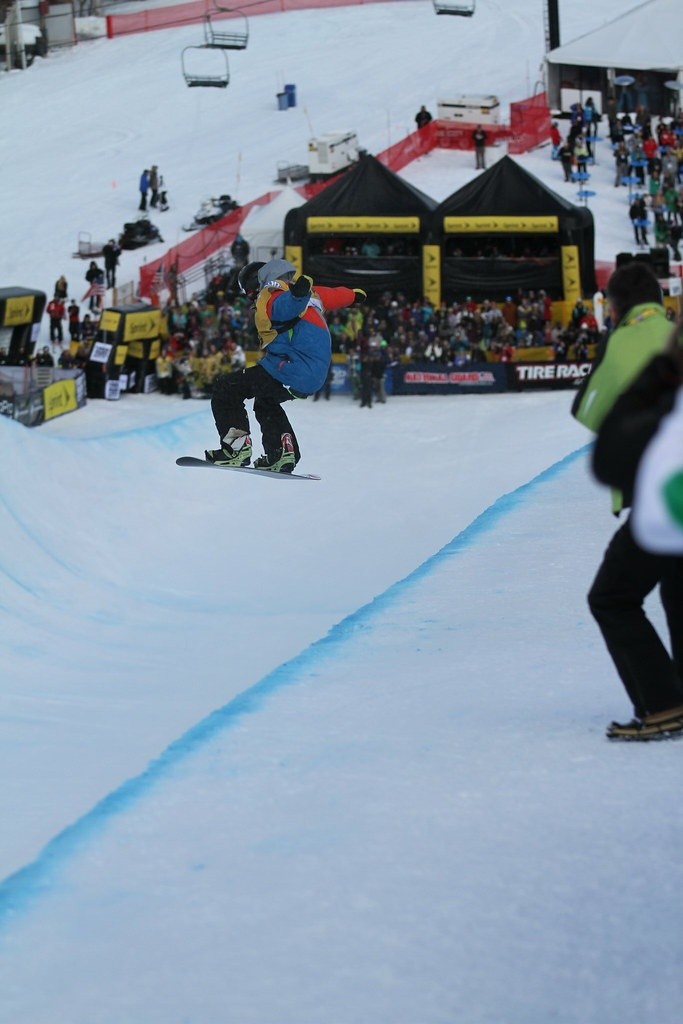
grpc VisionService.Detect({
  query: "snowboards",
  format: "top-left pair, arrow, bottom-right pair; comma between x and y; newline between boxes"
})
175,456 -> 321,480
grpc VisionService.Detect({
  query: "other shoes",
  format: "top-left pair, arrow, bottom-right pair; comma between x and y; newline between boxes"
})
606,706 -> 683,740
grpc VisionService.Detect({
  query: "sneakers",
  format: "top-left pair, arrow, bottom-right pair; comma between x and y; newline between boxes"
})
205,446 -> 251,466
253,448 -> 293,471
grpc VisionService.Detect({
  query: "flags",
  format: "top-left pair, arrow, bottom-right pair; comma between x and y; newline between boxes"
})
81,275 -> 104,301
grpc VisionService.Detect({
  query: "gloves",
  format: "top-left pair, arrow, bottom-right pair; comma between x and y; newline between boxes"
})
288,276 -> 311,298
354,289 -> 368,302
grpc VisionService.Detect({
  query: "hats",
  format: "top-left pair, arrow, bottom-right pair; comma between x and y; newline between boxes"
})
608,263 -> 662,319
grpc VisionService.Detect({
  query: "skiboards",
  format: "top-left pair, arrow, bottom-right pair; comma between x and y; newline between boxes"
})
52,343 -> 65,354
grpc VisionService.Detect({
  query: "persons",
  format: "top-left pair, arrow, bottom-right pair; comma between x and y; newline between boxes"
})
47,294 -> 63,343
570,263 -> 679,519
102,239 -> 121,289
231,235 -> 250,265
79,314 -> 96,339
314,290 -> 612,407
587,321 -> 683,742
149,165 -> 159,207
550,96 -> 683,244
206,259 -> 367,472
0,346 -> 72,369
55,276 -> 68,303
68,300 -> 80,339
86,260 -> 104,311
416,106 -> 432,129
155,273 -> 260,399
164,264 -> 180,306
472,124 -> 487,169
138,170 -> 149,210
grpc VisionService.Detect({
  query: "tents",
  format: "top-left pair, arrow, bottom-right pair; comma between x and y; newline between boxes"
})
284,154 -> 598,302
546,0 -> 683,110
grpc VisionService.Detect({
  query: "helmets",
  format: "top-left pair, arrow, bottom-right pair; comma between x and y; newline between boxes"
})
238,261 -> 266,291
